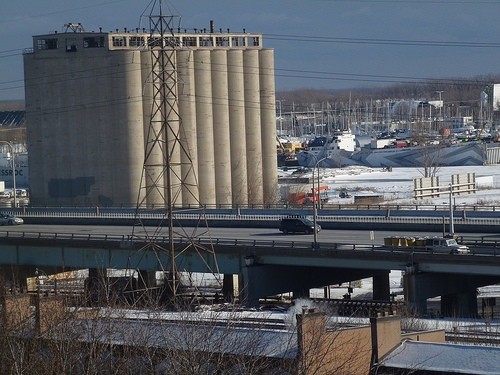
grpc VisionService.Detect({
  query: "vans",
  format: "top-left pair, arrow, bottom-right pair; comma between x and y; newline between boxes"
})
279,218 -> 321,235
15,189 -> 26,197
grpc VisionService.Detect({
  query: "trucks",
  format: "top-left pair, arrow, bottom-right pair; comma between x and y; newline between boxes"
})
305,192 -> 327,203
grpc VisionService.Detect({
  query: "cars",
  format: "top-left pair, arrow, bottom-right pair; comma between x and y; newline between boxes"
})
0,213 -> 24,226
0,189 -> 13,198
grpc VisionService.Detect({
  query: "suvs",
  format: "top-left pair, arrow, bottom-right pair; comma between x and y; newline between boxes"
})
432,238 -> 468,256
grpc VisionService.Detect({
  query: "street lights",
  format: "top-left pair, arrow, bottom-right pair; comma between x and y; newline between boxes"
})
313,155 -> 333,242
303,151 -> 320,210
0,141 -> 17,208
